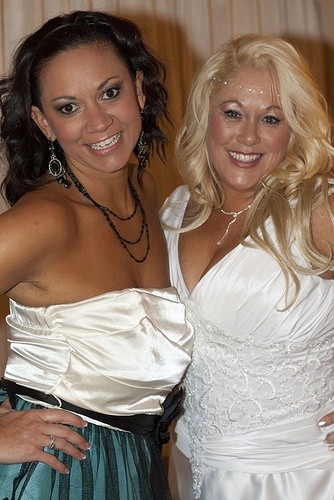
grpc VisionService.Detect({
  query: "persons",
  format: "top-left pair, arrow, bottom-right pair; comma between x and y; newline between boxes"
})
0,9 -> 195,500
157,32 -> 334,500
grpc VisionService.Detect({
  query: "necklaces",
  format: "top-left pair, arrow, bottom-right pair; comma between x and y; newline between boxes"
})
216,204 -> 251,244
62,158 -> 150,263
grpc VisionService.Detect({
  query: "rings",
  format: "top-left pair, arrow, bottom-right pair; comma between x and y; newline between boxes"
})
48,435 -> 58,450
